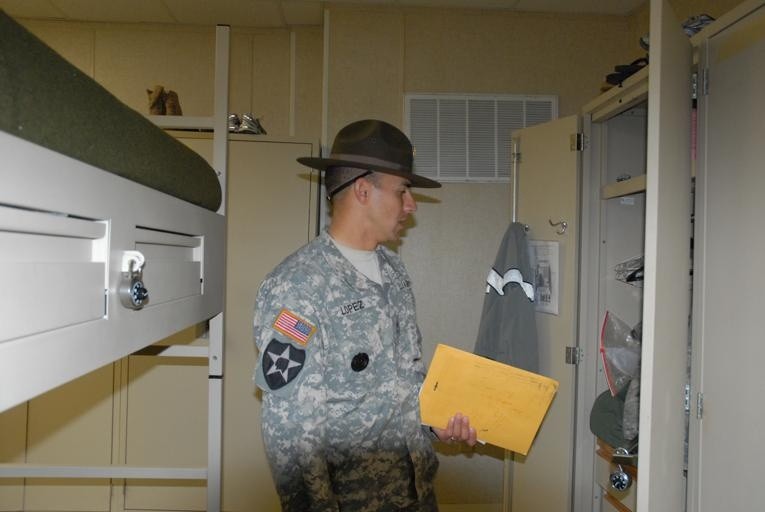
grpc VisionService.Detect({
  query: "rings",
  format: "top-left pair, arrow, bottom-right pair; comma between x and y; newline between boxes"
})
450,437 -> 457,441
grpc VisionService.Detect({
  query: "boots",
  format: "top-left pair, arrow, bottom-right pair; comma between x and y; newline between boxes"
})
146,85 -> 183,116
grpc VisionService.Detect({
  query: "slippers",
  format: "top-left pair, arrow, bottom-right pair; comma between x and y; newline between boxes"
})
606,57 -> 648,83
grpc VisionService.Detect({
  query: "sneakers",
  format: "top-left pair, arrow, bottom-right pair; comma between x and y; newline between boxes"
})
229,112 -> 267,136
639,13 -> 716,50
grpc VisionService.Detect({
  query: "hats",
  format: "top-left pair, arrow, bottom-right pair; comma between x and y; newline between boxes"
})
297,119 -> 442,188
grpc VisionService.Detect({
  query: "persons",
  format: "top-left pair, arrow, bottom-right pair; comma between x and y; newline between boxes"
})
252,120 -> 476,512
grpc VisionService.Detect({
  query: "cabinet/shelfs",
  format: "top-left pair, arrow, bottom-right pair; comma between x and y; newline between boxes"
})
0,130 -> 320,512
504,3 -> 764,510
